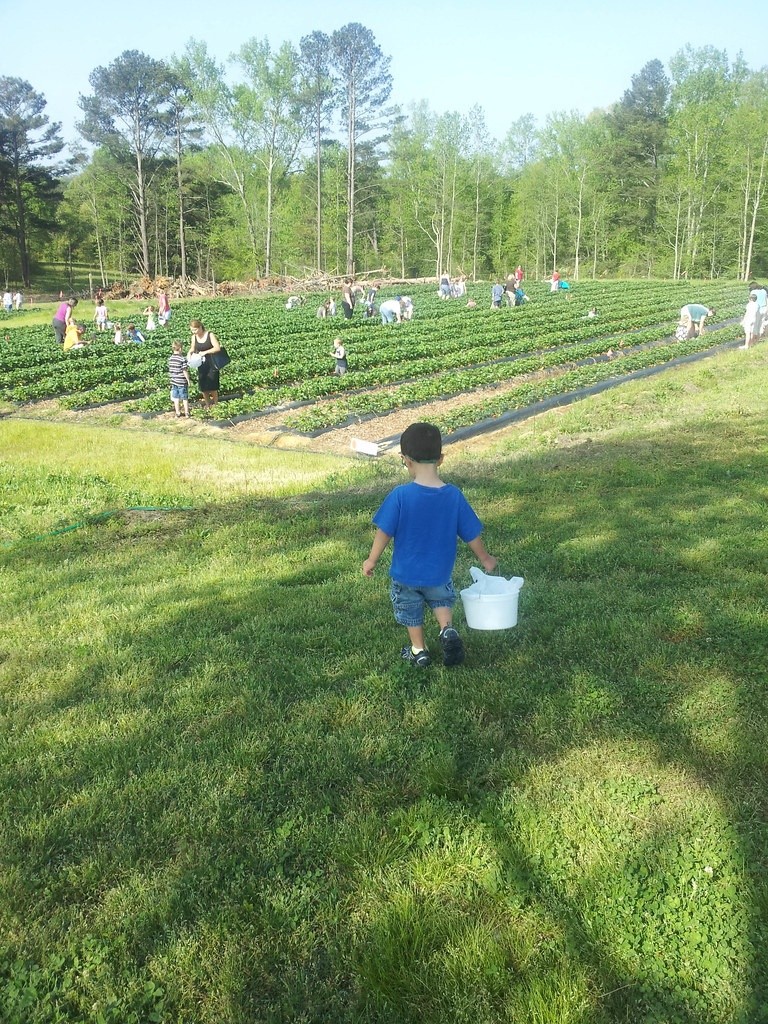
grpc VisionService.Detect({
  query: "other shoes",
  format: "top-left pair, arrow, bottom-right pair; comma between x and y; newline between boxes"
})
739,345 -> 750,351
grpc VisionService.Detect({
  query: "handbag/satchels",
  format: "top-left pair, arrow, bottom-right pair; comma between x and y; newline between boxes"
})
208,331 -> 231,370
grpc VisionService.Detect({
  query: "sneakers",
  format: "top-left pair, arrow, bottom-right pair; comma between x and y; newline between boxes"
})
440,628 -> 465,665
401,646 -> 431,667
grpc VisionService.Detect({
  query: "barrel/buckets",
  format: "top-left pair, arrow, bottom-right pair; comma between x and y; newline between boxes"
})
459,562 -> 519,630
107,320 -> 114,330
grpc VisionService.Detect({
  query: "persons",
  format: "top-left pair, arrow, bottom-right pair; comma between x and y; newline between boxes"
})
551,272 -> 558,291
143,306 -> 157,330
363,422 -> 496,666
380,296 -> 413,325
169,338 -> 191,418
491,279 -> 505,307
126,323 -> 145,343
288,295 -> 307,306
676,304 -> 716,340
341,277 -> 365,320
317,296 -> 336,318
4,289 -> 24,312
114,322 -> 123,344
93,298 -> 109,331
516,265 -> 523,281
505,274 -> 520,307
589,307 -> 598,317
330,338 -> 347,374
64,323 -> 91,351
156,288 -> 171,328
441,269 -> 467,300
187,320 -> 221,406
52,298 -> 78,343
367,285 -> 380,315
739,282 -> 768,350
